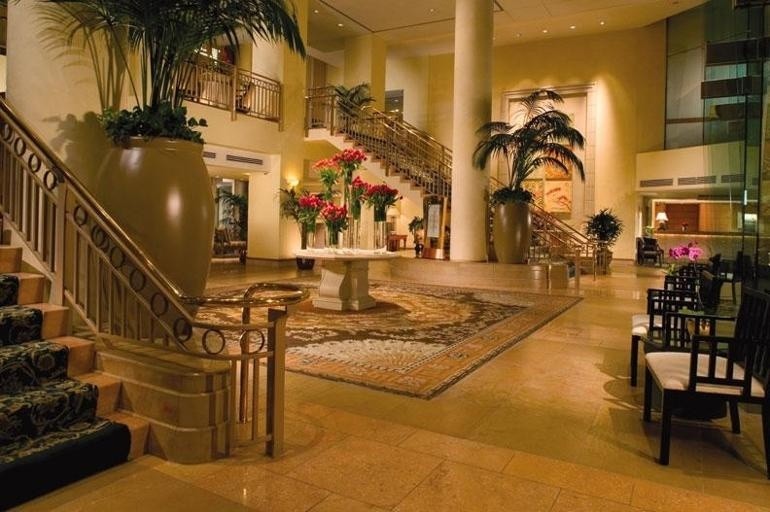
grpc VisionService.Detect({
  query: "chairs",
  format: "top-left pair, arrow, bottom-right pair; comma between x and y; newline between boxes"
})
233,81 -> 257,114
629,252 -> 770,479
635,236 -> 664,268
213,228 -> 248,265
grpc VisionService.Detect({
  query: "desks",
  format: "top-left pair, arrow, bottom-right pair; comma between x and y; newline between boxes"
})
290,243 -> 400,312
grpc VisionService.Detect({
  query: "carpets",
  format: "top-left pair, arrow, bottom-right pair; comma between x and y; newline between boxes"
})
185,274 -> 585,402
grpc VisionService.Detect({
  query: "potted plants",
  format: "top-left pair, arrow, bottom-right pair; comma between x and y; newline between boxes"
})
580,205 -> 626,267
35,1 -> 309,345
470,88 -> 589,266
408,214 -> 425,258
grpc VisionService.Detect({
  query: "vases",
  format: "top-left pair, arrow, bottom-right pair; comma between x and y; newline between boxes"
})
299,223 -> 389,254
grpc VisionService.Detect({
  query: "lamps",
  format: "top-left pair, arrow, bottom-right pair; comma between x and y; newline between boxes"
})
655,212 -> 669,232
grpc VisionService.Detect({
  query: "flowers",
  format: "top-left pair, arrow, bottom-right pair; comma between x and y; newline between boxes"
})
278,147 -> 401,244
